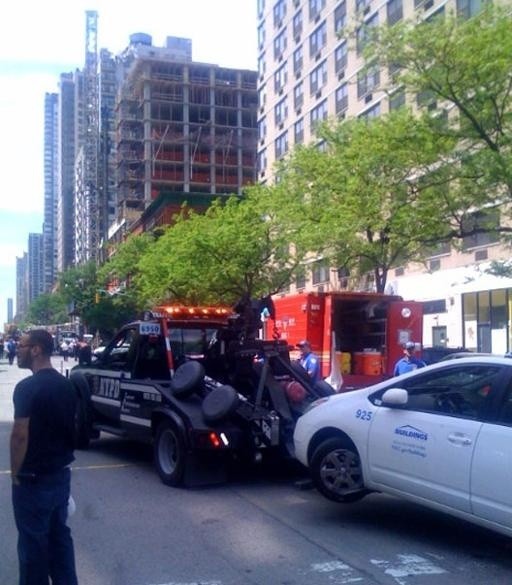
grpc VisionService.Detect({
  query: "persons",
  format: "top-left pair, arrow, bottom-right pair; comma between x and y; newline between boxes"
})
10,329 -> 78,584
393,341 -> 427,377
0,330 -> 23,365
51,335 -> 102,361
296,341 -> 319,385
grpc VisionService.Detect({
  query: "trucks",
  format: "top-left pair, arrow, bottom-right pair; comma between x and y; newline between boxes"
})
260,293 -> 425,392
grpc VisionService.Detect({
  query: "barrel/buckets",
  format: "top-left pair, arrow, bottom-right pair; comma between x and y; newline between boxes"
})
352,350 -> 364,373
364,351 -> 382,375
337,351 -> 352,373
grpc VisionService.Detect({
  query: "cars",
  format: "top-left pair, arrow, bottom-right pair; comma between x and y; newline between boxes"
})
66,303 -> 340,489
292,356 -> 512,534
438,352 -> 512,395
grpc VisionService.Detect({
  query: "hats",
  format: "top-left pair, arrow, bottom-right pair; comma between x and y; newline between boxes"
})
295,341 -> 309,347
403,341 -> 415,349
